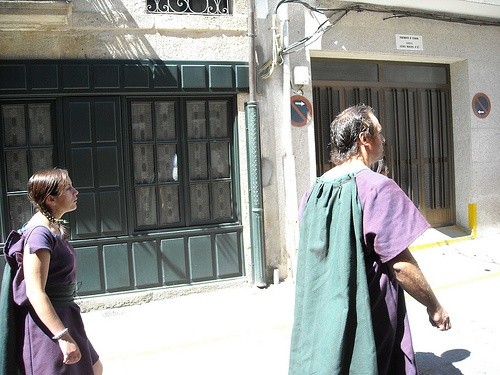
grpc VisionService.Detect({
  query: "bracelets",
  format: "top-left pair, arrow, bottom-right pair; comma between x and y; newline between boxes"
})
52,327 -> 68,340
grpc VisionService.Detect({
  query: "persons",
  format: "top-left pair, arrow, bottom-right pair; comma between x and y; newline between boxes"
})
288,102 -> 452,375
0,167 -> 104,375
